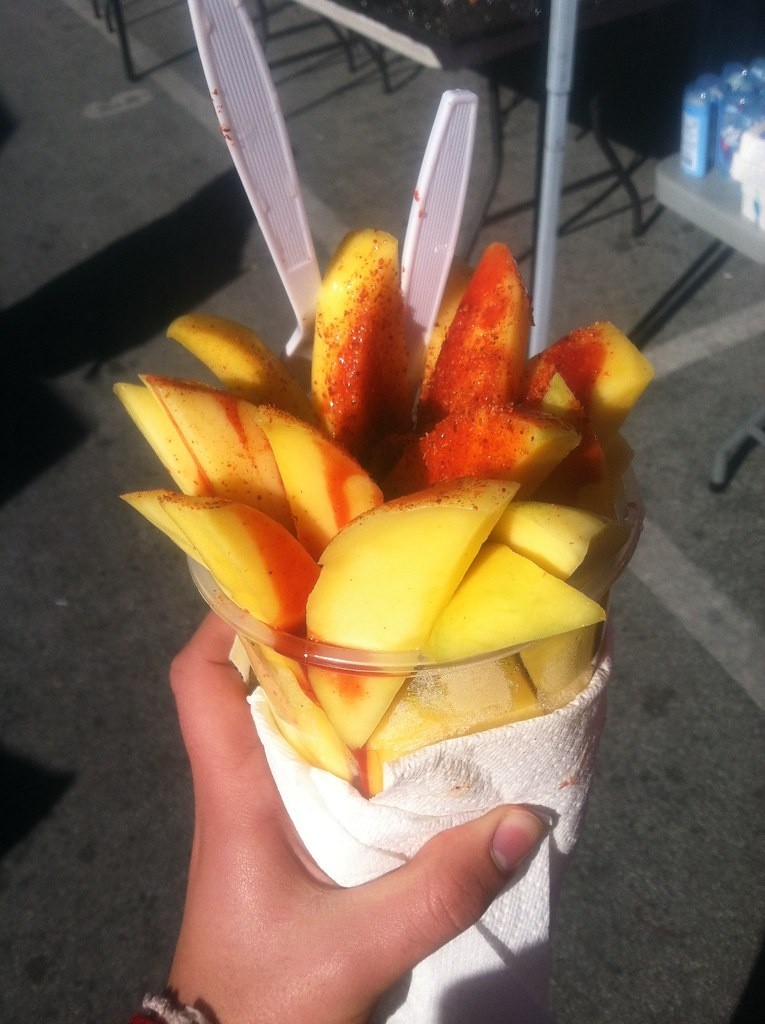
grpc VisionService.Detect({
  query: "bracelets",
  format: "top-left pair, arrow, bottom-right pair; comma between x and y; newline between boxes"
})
129,992 -> 210,1024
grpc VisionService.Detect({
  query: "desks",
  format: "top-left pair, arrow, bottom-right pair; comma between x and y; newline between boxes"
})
100,0 -> 764,492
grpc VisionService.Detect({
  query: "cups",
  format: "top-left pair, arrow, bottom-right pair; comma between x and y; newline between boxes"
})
189,494 -> 645,802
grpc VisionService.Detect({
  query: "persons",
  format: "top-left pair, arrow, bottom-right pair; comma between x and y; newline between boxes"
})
129,610 -> 543,1024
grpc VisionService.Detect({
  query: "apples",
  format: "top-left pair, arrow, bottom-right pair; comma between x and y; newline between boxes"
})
109,227 -> 657,799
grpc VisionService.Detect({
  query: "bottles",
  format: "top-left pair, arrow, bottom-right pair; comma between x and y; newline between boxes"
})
678,49 -> 765,180
